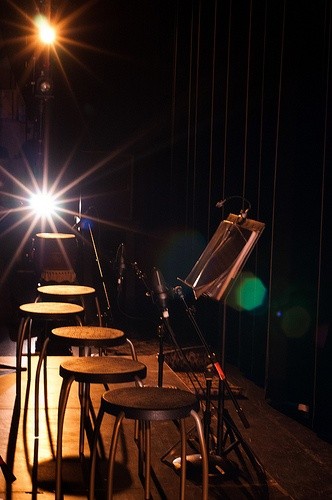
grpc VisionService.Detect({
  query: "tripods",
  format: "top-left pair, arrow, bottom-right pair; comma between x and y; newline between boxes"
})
160,215 -> 269,500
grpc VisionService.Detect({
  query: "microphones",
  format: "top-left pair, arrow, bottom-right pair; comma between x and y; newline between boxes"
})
155,271 -> 169,318
118,243 -> 124,284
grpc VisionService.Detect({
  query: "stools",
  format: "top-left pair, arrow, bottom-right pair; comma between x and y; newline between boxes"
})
27,285 -> 102,408
16,302 -> 85,466
55,357 -> 147,500
89,387 -> 208,500
32,326 -> 140,500
